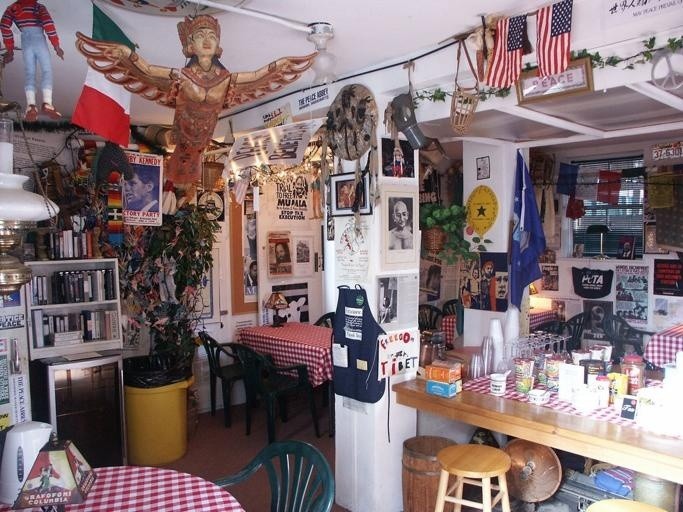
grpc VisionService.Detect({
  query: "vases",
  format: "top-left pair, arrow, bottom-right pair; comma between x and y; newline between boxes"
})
118,372 -> 197,468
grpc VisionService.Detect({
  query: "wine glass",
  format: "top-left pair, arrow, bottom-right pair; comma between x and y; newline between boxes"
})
504,330 -> 571,366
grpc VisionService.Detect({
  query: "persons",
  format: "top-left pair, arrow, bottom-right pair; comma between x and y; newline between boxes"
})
246,260 -> 257,286
76,146 -> 90,171
125,165 -> 159,213
389,200 -> 413,251
1,1 -> 65,121
275,242 -> 291,273
35,454 -> 84,493
538,241 -> 649,336
295,177 -> 305,194
309,167 -> 324,220
338,184 -> 356,208
380,297 -> 391,324
478,159 -> 489,177
393,144 -> 404,177
460,260 -> 509,309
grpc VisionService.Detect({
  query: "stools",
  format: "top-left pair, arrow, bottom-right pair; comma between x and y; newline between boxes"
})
432,442 -> 512,511
583,495 -> 665,512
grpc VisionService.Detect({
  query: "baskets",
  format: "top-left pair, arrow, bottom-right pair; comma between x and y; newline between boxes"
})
450,91 -> 479,136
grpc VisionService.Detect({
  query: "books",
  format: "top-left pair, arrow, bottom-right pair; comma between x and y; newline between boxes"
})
30,225 -> 120,348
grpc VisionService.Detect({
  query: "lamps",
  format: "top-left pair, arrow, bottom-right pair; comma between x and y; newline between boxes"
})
302,24 -> 342,91
9,432 -> 96,510
263,291 -> 289,328
528,283 -> 538,309
0,119 -> 61,303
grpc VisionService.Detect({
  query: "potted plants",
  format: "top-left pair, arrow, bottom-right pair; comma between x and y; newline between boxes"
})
414,200 -> 490,265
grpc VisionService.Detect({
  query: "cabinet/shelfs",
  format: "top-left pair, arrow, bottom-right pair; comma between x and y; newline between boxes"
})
22,256 -> 124,362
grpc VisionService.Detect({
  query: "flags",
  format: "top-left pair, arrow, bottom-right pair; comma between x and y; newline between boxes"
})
511,149 -> 547,313
556,162 -> 577,195
482,11 -> 528,89
535,1 -> 575,78
575,165 -> 599,202
597,170 -> 621,205
69,5 -> 135,149
234,181 -> 249,206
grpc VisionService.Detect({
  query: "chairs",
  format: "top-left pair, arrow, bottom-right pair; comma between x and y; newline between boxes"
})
417,298 -> 683,363
213,438 -> 334,511
193,332 -> 320,446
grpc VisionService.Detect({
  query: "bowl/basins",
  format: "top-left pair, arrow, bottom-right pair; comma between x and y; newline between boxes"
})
527,388 -> 550,405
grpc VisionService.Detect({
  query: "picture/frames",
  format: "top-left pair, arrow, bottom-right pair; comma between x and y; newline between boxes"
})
326,169 -> 371,216
509,56 -> 593,106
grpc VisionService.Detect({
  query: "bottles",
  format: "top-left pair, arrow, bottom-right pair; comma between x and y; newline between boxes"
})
596,375 -> 609,409
622,355 -> 646,394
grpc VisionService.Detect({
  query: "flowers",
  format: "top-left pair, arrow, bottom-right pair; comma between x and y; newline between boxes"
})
108,203 -> 218,380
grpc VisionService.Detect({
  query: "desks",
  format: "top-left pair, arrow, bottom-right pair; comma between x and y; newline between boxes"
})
0,464 -> 242,512
239,320 -> 333,438
391,345 -> 683,511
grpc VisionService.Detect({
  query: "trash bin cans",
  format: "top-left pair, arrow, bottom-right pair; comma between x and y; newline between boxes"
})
123,353 -> 195,466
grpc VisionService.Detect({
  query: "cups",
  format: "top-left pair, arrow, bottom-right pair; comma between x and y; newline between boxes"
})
468,309 -> 520,379
572,343 -> 613,365
489,373 -> 507,395
